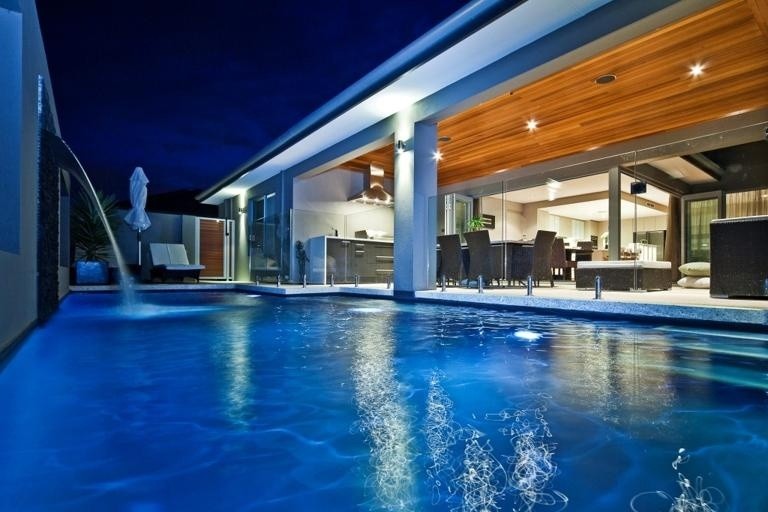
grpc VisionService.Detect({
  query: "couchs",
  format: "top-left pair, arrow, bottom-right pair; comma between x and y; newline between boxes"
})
711,214 -> 766,299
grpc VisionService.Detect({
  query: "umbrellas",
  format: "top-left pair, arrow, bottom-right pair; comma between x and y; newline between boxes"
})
122,167 -> 152,280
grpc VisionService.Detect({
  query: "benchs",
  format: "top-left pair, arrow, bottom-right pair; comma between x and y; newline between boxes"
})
575,260 -> 673,291
150,243 -> 205,284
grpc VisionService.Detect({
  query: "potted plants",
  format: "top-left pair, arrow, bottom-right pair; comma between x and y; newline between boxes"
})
70,188 -> 122,285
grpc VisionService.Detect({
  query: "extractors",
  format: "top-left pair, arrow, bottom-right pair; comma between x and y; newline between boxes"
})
346,162 -> 394,206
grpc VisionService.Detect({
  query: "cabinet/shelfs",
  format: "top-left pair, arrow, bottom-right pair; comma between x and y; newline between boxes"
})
634,231 -> 666,261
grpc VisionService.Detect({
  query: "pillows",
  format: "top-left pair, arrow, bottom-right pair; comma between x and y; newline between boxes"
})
677,261 -> 711,289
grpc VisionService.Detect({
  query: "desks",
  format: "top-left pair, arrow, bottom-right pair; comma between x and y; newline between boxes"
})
300,235 -> 395,288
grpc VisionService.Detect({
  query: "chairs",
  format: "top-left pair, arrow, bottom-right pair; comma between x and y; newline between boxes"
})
434,229 -> 593,296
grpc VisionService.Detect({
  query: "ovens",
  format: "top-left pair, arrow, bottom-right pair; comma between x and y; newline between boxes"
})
375,246 -> 393,282
